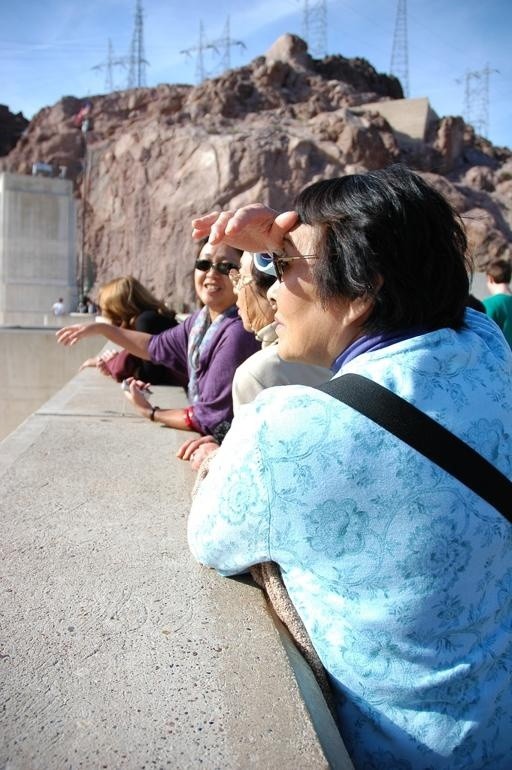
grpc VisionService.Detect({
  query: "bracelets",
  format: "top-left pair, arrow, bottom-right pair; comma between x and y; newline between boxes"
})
148,404 -> 160,422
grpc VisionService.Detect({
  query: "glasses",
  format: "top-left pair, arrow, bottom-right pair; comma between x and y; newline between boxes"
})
272,251 -> 322,284
195,259 -> 238,277
228,268 -> 259,287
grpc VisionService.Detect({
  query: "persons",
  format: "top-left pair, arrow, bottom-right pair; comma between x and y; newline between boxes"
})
54,233 -> 262,438
184,158 -> 512,770
49,274 -> 192,393
483,256 -> 511,348
175,251 -> 335,471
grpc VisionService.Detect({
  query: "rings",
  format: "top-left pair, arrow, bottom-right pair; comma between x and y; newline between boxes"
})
190,451 -> 197,461
